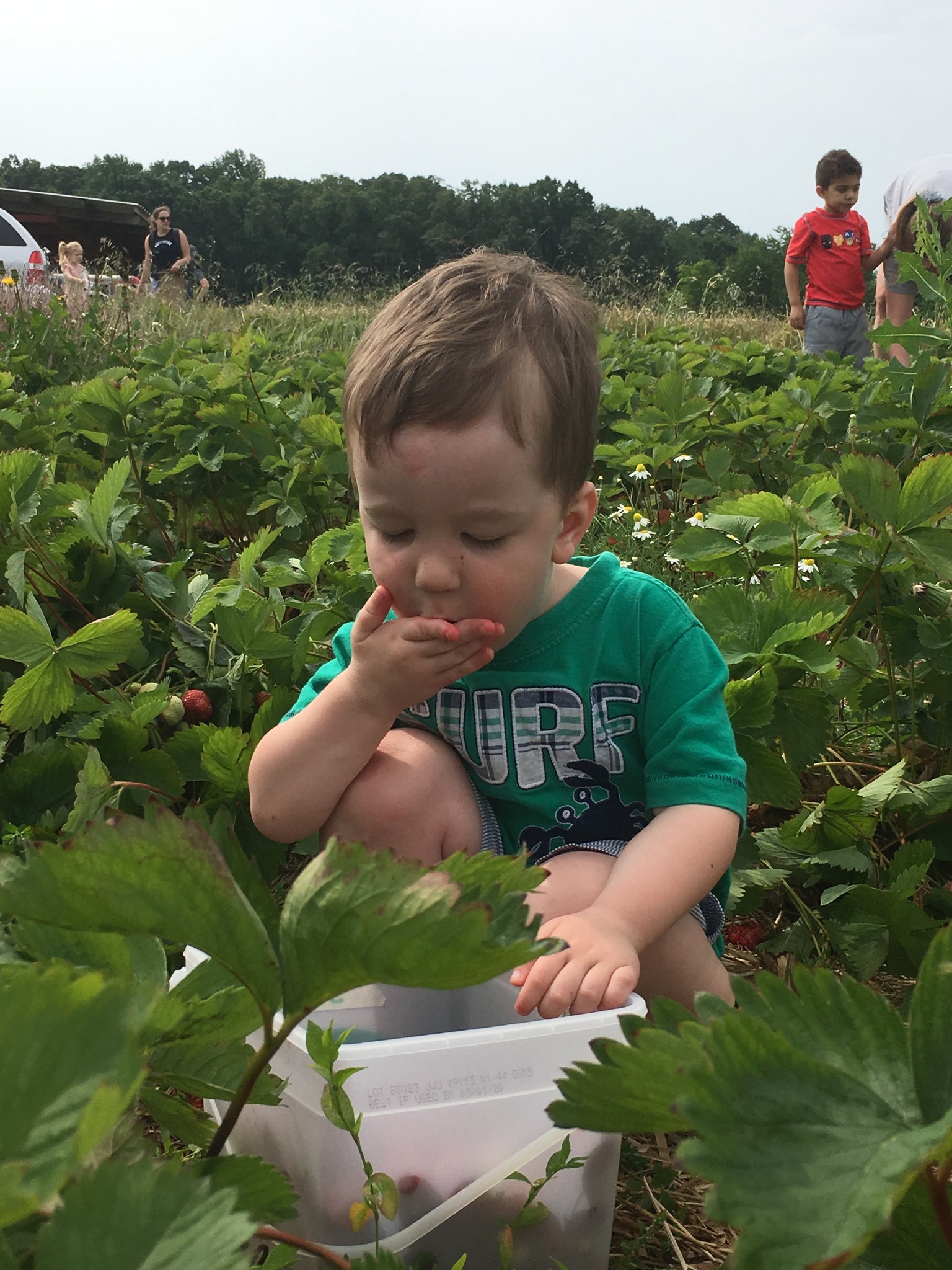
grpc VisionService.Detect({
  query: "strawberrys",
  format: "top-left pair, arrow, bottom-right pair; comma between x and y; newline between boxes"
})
0,508 -> 952,1270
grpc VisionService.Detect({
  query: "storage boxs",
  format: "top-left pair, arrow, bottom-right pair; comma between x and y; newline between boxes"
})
156,939 -> 652,1270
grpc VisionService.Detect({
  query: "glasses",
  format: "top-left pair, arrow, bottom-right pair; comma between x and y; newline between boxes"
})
76,253 -> 82,256
159,216 -> 171,222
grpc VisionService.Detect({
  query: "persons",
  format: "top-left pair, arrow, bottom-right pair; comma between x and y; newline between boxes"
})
58,240 -> 89,322
784,148 -> 898,374
138,205 -> 191,308
185,244 -> 209,304
871,154 -> 952,369
248,246 -> 745,1018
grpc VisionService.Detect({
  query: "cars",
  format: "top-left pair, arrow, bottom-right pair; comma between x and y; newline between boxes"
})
0,207 -> 48,295
